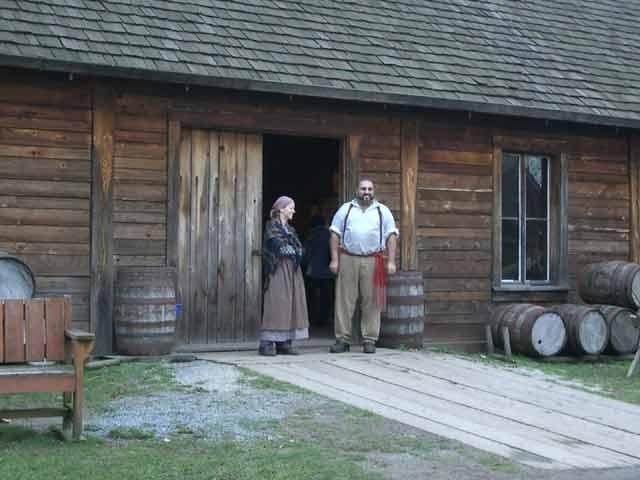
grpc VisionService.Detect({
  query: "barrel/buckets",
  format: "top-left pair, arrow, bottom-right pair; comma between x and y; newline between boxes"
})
114,265 -> 177,355
486,303 -> 568,357
377,270 -> 425,347
578,260 -> 640,305
602,303 -> 640,353
551,303 -> 608,354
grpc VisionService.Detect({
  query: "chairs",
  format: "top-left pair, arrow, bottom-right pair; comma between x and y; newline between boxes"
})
0,292 -> 97,446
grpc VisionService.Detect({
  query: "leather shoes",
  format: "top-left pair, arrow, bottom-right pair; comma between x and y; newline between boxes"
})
276,344 -> 300,355
330,340 -> 350,353
363,342 -> 376,353
259,345 -> 276,356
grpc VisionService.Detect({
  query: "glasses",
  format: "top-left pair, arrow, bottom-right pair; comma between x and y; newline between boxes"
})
361,187 -> 372,191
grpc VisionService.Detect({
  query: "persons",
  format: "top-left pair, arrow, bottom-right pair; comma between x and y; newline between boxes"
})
308,204 -> 322,217
258,196 -> 310,356
301,214 -> 334,327
325,179 -> 400,354
